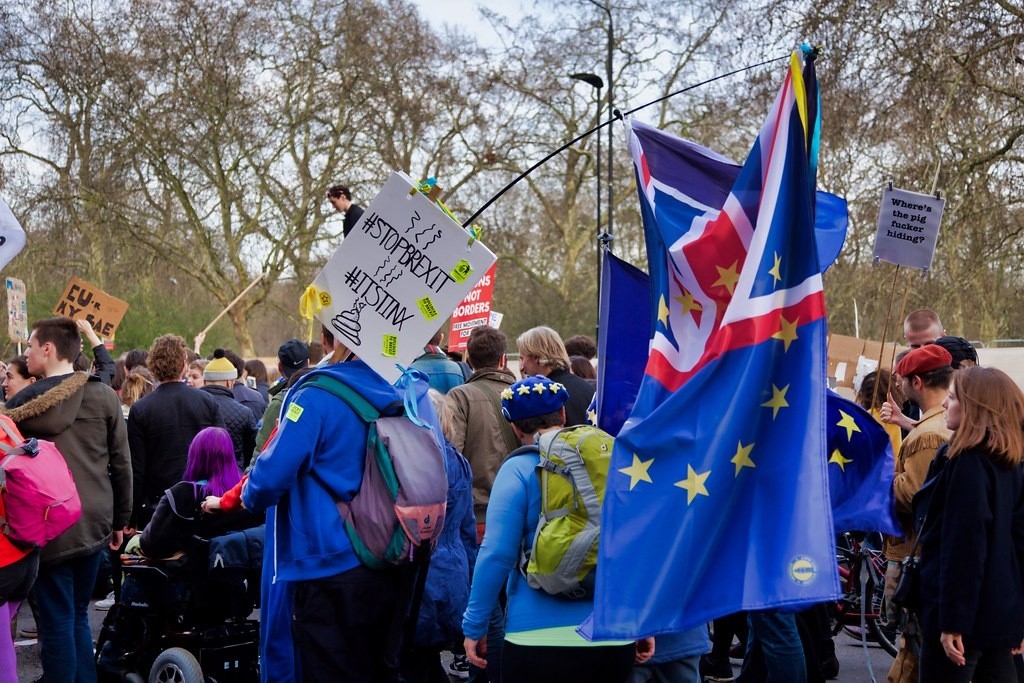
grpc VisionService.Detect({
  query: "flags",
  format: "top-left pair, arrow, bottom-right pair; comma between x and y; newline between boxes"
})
577,42 -> 900,641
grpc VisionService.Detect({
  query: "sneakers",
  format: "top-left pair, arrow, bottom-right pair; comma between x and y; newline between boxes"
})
448,657 -> 470,678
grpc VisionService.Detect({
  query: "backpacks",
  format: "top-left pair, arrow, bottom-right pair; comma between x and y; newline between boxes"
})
0,415 -> 82,547
501,423 -> 616,593
297,374 -> 450,562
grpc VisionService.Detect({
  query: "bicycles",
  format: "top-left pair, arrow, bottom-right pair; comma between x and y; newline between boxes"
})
830,530 -> 901,657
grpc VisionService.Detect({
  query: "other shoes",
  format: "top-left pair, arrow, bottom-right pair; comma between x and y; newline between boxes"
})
728,641 -> 747,660
19,625 -> 38,636
94,590 -> 115,609
703,657 -> 734,681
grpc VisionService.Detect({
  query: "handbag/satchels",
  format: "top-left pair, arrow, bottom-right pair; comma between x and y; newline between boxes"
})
891,555 -> 924,610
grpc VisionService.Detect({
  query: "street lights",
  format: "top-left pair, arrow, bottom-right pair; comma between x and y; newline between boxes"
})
572,72 -> 601,342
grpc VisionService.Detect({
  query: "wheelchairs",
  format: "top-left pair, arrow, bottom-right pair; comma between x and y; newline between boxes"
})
93,499 -> 267,683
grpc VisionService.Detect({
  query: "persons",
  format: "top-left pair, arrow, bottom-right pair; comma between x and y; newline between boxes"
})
0,318 -> 842,683
853,299 -> 1024,683
327,184 -> 364,238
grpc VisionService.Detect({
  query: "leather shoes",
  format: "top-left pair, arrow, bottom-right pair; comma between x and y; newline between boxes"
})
843,624 -> 895,645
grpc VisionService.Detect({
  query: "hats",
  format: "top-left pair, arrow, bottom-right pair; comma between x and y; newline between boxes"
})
895,345 -> 952,378
500,375 -> 566,422
204,349 -> 238,380
278,338 -> 309,376
936,336 -> 977,360
